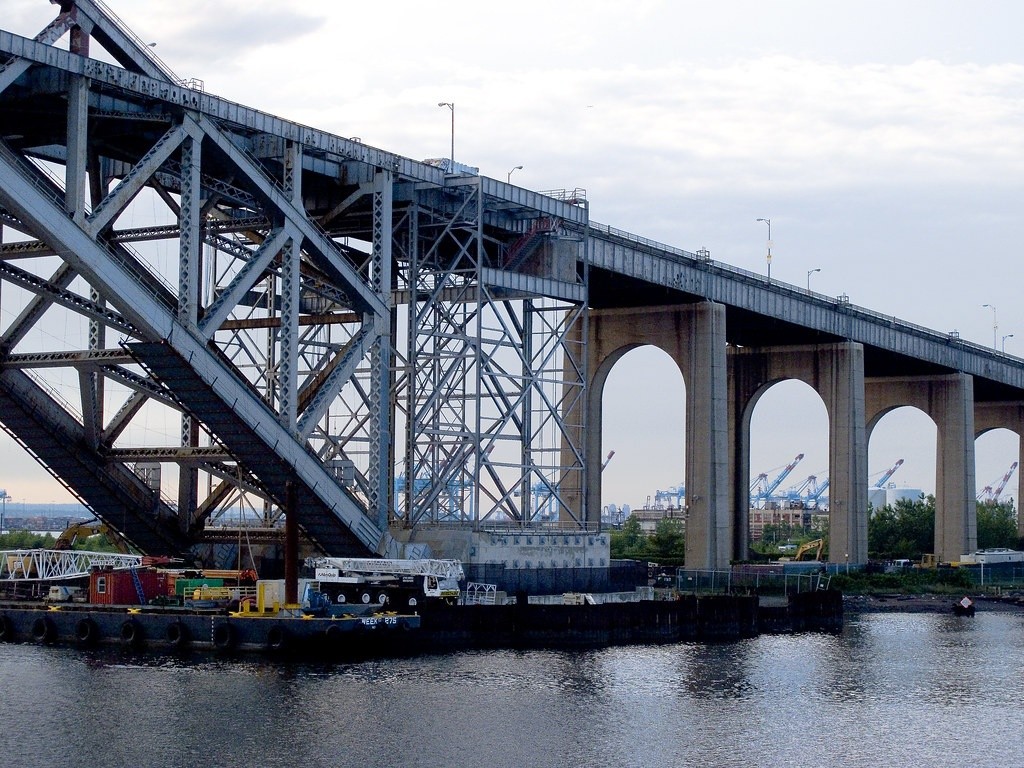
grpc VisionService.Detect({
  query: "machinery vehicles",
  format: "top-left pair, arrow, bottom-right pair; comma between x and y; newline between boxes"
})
303,552 -> 465,606
975,461 -> 1018,502
867,458 -> 906,488
651,454 -> 829,511
779,538 -> 824,561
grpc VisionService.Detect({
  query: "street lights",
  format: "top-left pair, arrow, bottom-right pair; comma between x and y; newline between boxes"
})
1002,334 -> 1014,357
807,268 -> 821,290
508,166 -> 523,183
756,218 -> 770,282
438,101 -> 455,174
983,304 -> 997,353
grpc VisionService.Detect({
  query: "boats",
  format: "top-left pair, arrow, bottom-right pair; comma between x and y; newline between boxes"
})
1,547 -> 423,655
